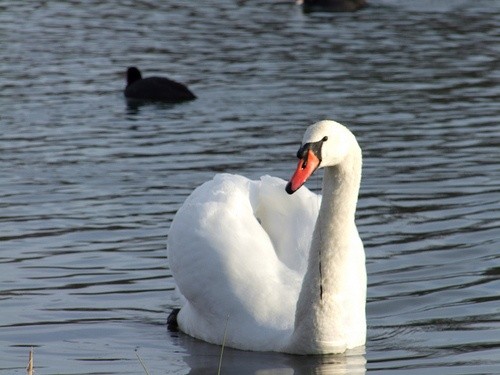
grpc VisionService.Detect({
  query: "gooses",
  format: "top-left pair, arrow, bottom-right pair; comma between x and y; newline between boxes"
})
166,119 -> 369,354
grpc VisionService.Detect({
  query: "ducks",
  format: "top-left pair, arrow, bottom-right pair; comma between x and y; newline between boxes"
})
125,67 -> 195,105
